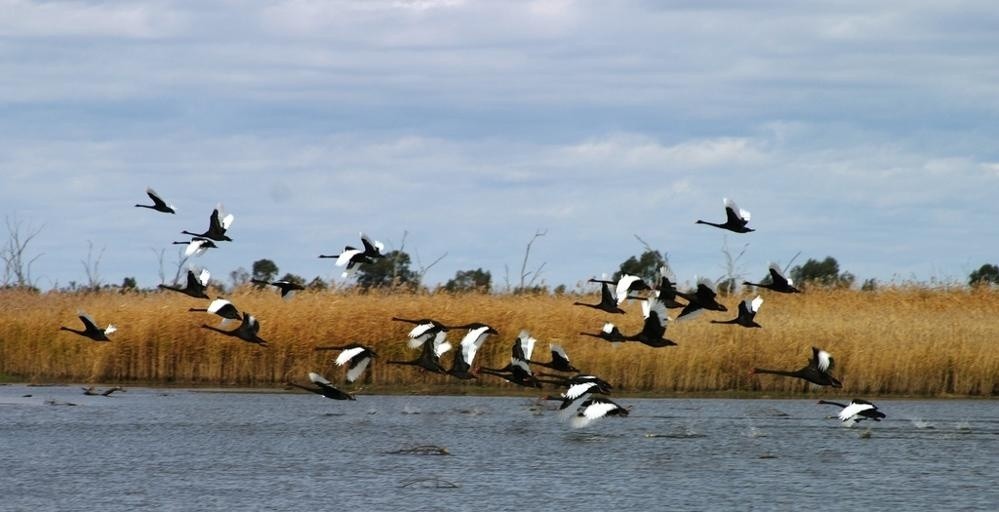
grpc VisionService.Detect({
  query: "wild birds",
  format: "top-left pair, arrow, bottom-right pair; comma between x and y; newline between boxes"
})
135,187 -> 176,215
197,312 -> 267,343
250,277 -> 305,302
750,346 -> 843,388
171,237 -> 218,257
316,232 -> 388,279
817,398 -> 886,422
693,197 -> 757,234
284,317 -> 630,420
180,203 -> 235,242
58,314 -> 119,343
574,269 -> 805,349
187,296 -> 244,322
155,269 -> 210,299
